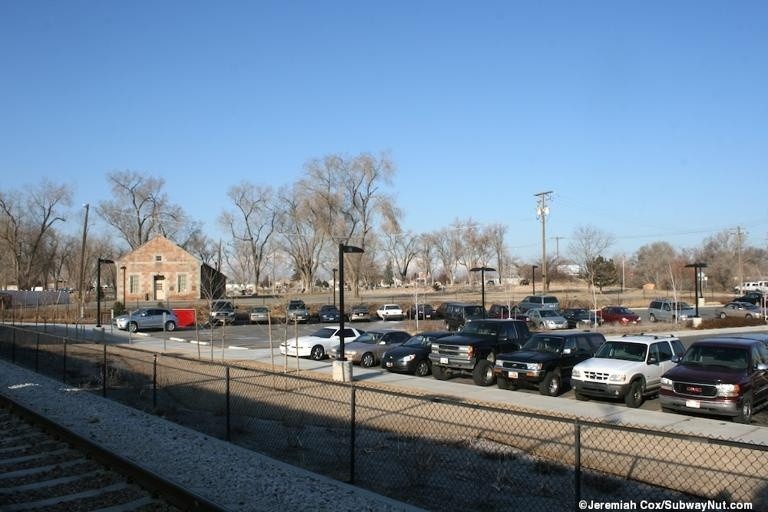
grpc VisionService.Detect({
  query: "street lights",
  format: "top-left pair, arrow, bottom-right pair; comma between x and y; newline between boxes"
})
684,263 -> 706,318
96,255 -> 114,327
337,241 -> 364,362
331,268 -> 338,306
533,190 -> 553,295
469,267 -> 496,320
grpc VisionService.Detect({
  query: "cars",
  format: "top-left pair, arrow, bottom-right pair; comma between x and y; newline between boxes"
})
114,305 -> 179,333
433,294 -> 642,330
278,318 -> 606,398
100,284 -> 107,290
656,333 -> 768,424
484,272 -> 527,285
571,336 -> 687,408
714,281 -> 768,323
209,298 -> 433,325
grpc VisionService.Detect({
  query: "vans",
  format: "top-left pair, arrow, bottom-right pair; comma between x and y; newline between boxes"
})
648,300 -> 697,323
